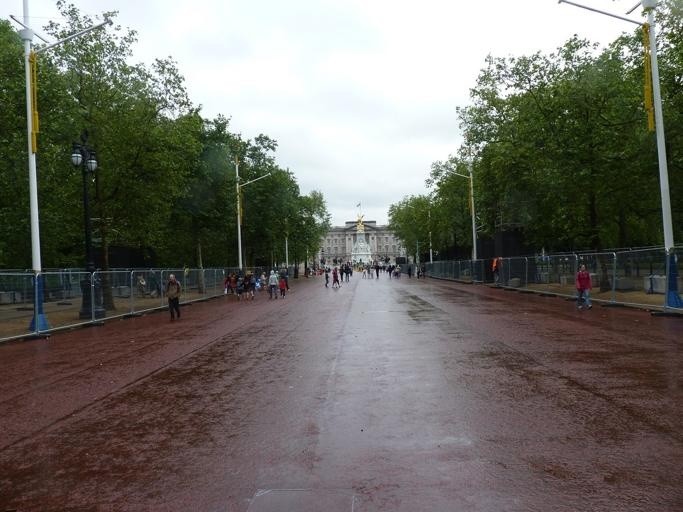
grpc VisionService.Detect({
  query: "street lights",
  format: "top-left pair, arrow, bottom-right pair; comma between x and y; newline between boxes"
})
70,130 -> 104,319
11,0 -> 113,331
557,0 -> 683,307
234,156 -> 271,269
440,158 -> 477,260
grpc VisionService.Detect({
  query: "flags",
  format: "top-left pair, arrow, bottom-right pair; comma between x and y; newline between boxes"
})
356,201 -> 361,208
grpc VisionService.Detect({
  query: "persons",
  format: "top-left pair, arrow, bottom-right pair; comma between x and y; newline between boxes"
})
574,261 -> 594,310
317,256 -> 425,290
221,267 -> 290,300
164,273 -> 182,323
